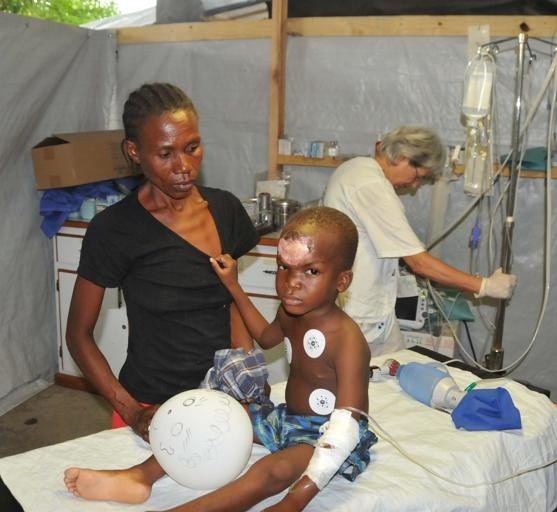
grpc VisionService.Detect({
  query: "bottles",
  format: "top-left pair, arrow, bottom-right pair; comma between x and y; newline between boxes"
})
461,47 -> 493,128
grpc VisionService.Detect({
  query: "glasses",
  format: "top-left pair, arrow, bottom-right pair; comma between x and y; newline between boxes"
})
413,163 -> 424,182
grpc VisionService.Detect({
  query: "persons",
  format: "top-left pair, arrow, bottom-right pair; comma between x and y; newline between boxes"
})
62,205 -> 378,512
66,83 -> 272,444
322,125 -> 518,359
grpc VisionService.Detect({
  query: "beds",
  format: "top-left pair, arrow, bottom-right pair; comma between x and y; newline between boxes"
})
0,346 -> 557,512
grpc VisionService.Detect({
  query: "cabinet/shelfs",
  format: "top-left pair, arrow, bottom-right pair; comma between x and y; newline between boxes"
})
53,224 -> 291,396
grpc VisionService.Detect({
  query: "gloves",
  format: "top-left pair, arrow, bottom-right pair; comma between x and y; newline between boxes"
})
473,267 -> 518,300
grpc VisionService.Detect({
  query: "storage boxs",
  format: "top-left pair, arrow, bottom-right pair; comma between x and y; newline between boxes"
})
30,129 -> 137,191
